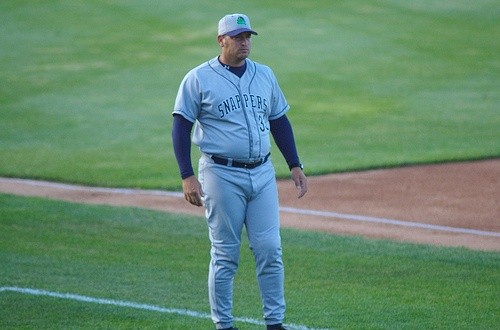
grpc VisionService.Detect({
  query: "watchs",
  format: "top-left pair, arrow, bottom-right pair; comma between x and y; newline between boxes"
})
289,162 -> 304,172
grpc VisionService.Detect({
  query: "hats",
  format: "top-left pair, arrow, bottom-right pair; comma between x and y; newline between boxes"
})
217,14 -> 258,37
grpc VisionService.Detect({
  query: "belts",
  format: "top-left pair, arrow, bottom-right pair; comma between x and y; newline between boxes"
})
211,152 -> 272,169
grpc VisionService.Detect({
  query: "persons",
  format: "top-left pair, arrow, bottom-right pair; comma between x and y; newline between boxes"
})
171,13 -> 308,330
